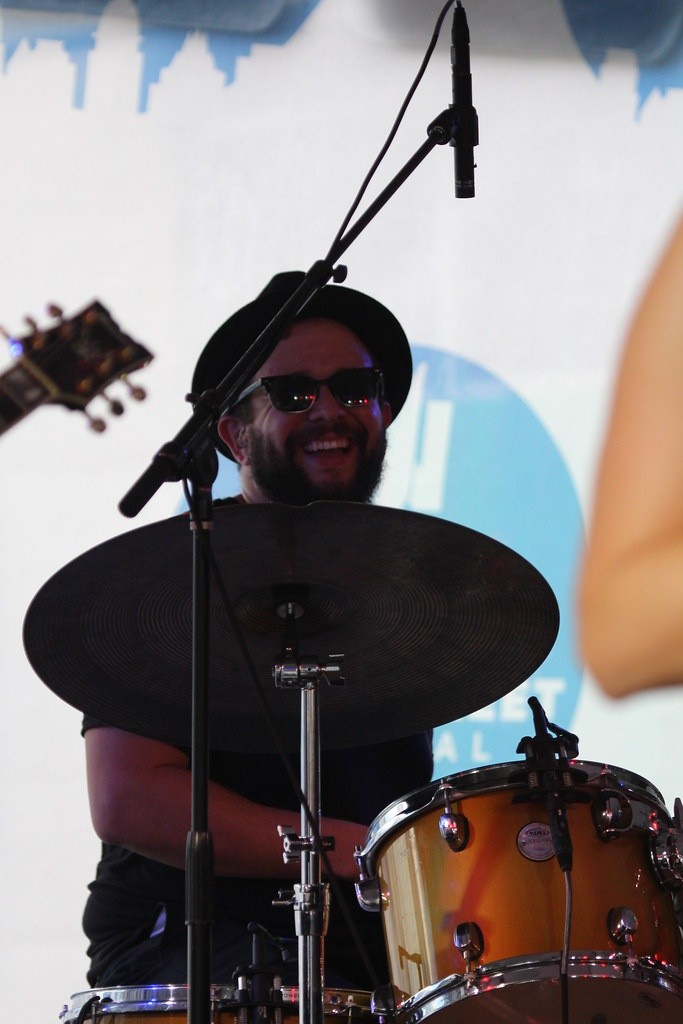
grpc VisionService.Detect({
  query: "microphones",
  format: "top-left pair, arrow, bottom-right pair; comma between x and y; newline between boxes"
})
450,7 -> 479,199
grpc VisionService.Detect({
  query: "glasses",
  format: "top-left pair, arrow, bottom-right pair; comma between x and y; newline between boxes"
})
222,368 -> 386,420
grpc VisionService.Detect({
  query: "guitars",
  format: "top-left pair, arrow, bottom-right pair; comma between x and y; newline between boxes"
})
1,301 -> 155,439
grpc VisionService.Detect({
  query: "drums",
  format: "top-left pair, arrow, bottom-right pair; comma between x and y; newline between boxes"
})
60,984 -> 371,1024
352,756 -> 683,1024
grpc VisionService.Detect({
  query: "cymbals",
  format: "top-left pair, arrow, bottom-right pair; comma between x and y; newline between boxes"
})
23,498 -> 561,759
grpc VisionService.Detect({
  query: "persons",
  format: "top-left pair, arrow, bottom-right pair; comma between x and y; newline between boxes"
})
82,272 -> 436,990
575,219 -> 683,697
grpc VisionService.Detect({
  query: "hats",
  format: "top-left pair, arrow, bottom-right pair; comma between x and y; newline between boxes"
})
191,271 -> 412,463
